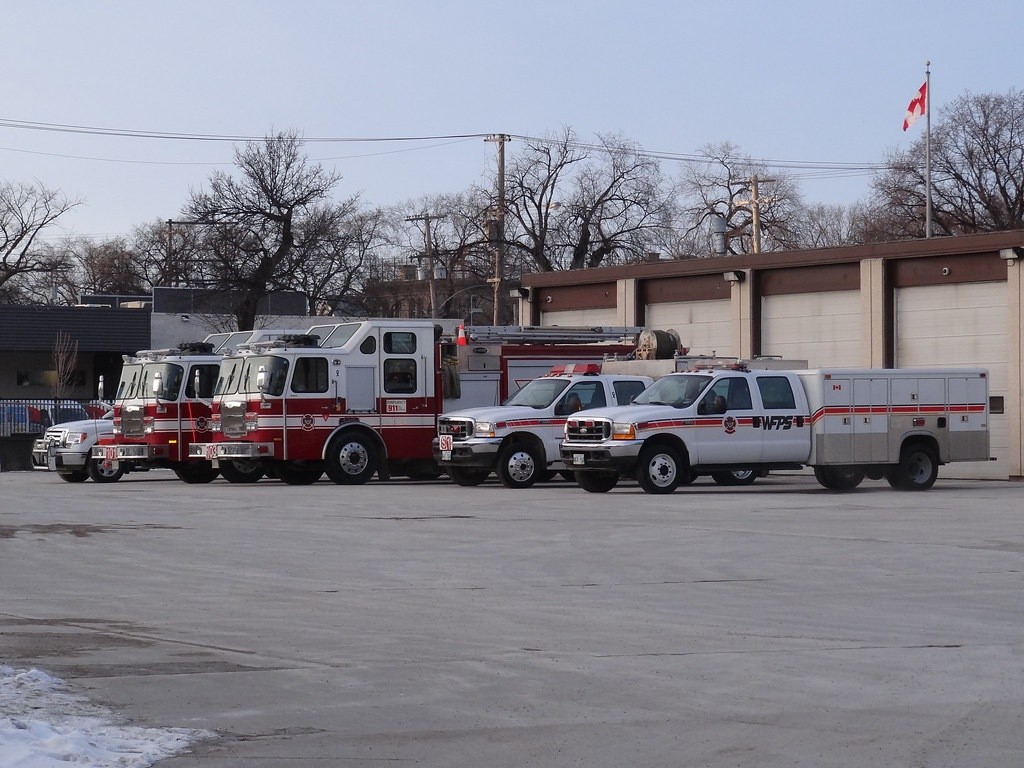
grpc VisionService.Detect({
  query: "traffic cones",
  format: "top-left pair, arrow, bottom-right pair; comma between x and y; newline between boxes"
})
457,324 -> 467,346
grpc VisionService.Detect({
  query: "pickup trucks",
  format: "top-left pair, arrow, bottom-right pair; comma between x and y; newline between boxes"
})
558,367 -> 990,494
432,363 -> 661,489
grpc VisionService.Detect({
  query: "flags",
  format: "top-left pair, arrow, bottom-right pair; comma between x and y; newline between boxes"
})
903,80 -> 926,129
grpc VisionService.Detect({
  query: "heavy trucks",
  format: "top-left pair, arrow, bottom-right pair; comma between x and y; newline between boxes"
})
32,321 -> 808,485
